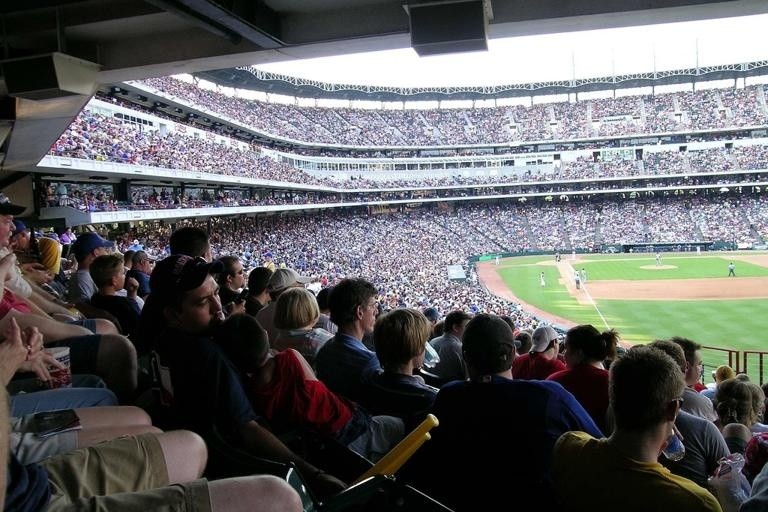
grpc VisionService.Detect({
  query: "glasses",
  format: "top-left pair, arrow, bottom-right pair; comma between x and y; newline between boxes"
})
362,301 -> 379,310
230,268 -> 244,277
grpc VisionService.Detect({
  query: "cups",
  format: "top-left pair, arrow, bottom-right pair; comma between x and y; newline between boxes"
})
34,347 -> 73,390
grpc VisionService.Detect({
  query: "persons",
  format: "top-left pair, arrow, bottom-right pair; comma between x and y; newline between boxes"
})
130,76 -> 767,158
0,189 -> 768,512
49,107 -> 767,211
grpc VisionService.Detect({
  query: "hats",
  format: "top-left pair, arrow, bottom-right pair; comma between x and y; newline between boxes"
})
149,254 -> 225,306
0,192 -> 27,216
12,221 -> 26,237
462,313 -> 515,357
73,232 -> 115,253
445,310 -> 472,322
530,325 -> 560,353
266,267 -> 312,294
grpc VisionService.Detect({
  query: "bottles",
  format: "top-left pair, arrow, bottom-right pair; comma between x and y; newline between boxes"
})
659,428 -> 686,462
422,340 -> 441,369
